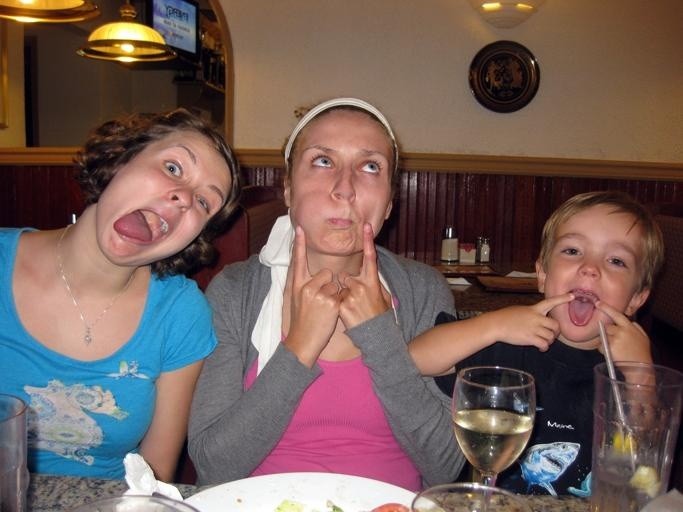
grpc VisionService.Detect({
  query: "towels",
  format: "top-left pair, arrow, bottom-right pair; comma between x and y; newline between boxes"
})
251,214 -> 294,377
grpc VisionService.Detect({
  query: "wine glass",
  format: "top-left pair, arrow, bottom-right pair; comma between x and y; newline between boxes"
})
450,364 -> 538,507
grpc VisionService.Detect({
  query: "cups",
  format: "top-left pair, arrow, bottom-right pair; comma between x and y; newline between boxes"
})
588,361 -> 683,512
1,392 -> 33,512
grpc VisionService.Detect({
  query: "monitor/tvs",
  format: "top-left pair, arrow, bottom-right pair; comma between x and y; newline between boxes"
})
146,0 -> 201,64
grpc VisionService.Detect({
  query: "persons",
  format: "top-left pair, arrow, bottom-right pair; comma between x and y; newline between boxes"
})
0,107 -> 253,489
186,95 -> 474,492
405,182 -> 668,501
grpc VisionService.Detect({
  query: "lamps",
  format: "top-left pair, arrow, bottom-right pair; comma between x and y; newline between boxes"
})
75,0 -> 178,63
470,0 -> 546,28
0,0 -> 101,23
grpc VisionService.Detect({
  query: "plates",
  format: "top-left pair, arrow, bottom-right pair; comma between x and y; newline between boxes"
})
180,470 -> 446,512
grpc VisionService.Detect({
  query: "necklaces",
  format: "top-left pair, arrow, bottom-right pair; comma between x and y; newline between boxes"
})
56,227 -> 138,346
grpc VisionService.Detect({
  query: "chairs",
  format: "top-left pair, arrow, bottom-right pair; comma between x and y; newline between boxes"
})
191,185 -> 279,295
638,210 -> 683,339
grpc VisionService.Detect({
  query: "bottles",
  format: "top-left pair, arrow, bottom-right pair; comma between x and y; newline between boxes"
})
440,224 -> 459,263
477,237 -> 491,263
